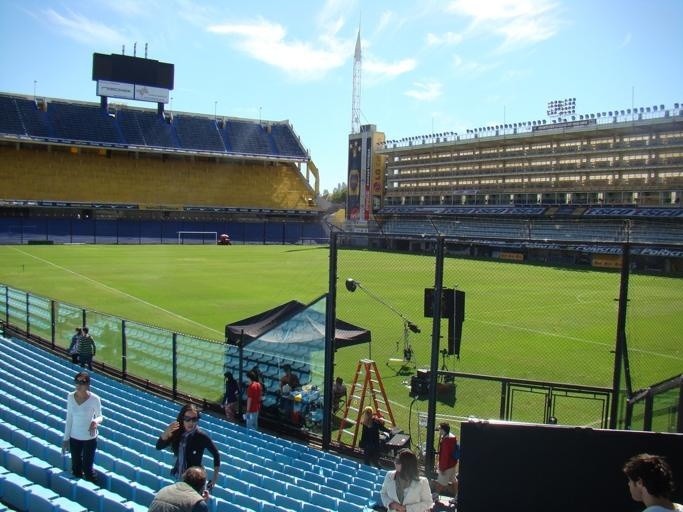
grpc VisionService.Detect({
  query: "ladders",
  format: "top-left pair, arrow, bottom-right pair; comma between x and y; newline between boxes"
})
337,359 -> 396,448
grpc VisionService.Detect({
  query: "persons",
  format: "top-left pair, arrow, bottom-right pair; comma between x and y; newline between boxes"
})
622,452 -> 682,512
155,402 -> 220,497
219,371 -> 240,423
358,406 -> 392,470
379,448 -> 434,512
244,370 -> 263,432
75,328 -> 96,371
147,465 -> 209,512
61,371 -> 104,483
331,377 -> 346,410
279,363 -> 300,424
430,420 -> 458,505
64,325 -> 82,364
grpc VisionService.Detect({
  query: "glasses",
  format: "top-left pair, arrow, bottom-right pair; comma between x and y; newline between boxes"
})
73,378 -> 86,385
393,457 -> 402,465
182,415 -> 199,423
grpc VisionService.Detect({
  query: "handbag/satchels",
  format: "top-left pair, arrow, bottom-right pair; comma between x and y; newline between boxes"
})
451,441 -> 461,461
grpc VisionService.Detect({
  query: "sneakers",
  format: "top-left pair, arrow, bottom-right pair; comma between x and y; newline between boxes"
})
432,492 -> 457,504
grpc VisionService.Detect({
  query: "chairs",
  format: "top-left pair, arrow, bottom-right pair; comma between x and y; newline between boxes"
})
0,285 -> 390,512
